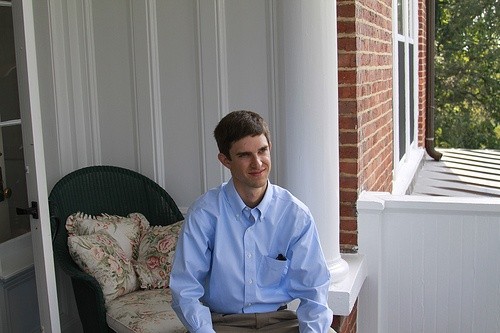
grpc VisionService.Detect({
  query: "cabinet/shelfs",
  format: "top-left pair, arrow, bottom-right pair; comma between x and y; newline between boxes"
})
1,230 -> 43,333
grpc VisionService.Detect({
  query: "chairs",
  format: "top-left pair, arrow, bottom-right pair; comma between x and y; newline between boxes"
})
50,165 -> 184,333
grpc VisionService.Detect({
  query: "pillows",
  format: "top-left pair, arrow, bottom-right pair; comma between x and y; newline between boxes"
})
131,219 -> 187,291
68,231 -> 142,304
66,213 -> 150,275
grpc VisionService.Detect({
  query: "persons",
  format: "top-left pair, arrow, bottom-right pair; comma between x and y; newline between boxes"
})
169,110 -> 338,333
